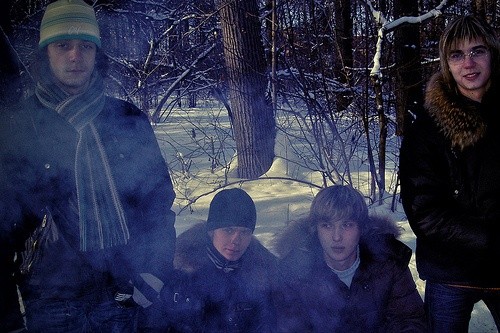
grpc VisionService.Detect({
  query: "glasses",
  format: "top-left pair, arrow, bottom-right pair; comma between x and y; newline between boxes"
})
446,48 -> 487,66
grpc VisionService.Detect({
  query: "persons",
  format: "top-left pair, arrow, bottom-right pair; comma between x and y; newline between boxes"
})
133,188 -> 278,333
399,16 -> 500,333
0,0 -> 179,333
266,185 -> 429,333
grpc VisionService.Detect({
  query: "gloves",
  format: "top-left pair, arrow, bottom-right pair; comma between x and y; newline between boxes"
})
115,273 -> 165,312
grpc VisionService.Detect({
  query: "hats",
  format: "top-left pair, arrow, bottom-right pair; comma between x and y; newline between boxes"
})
38,0 -> 101,49
207,188 -> 256,231
